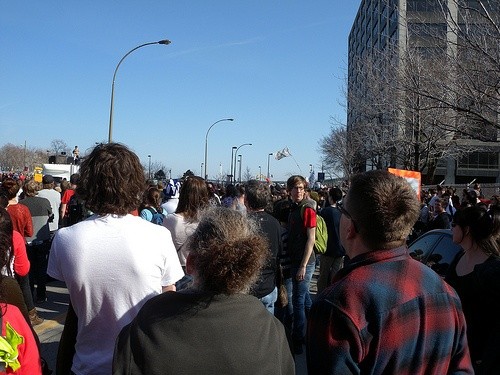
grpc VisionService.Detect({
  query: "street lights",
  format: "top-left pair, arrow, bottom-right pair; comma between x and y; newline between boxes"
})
109,39 -> 172,145
148,155 -> 151,178
231,143 -> 253,185
258,153 -> 273,178
201,119 -> 234,180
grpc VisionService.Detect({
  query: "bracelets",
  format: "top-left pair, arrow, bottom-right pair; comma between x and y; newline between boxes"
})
299,264 -> 307,267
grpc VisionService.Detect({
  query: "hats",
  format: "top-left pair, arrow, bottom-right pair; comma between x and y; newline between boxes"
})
70,173 -> 79,184
42,174 -> 54,184
474,185 -> 480,189
59,178 -> 69,183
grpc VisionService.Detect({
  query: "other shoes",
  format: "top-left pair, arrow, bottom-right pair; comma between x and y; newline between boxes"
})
292,342 -> 303,354
28,307 -> 44,326
36,291 -> 46,298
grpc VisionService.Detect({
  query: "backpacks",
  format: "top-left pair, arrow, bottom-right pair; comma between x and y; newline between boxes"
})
310,207 -> 328,254
147,206 -> 165,225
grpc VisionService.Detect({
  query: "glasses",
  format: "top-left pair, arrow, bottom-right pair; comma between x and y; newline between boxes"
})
435,203 -> 440,206
336,195 -> 354,221
451,223 -> 456,228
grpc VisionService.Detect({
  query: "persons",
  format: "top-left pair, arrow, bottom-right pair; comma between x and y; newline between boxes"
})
0,172 -> 500,375
47,142 -> 186,375
111,199 -> 296,375
73,145 -> 80,165
306,167 -> 476,375
